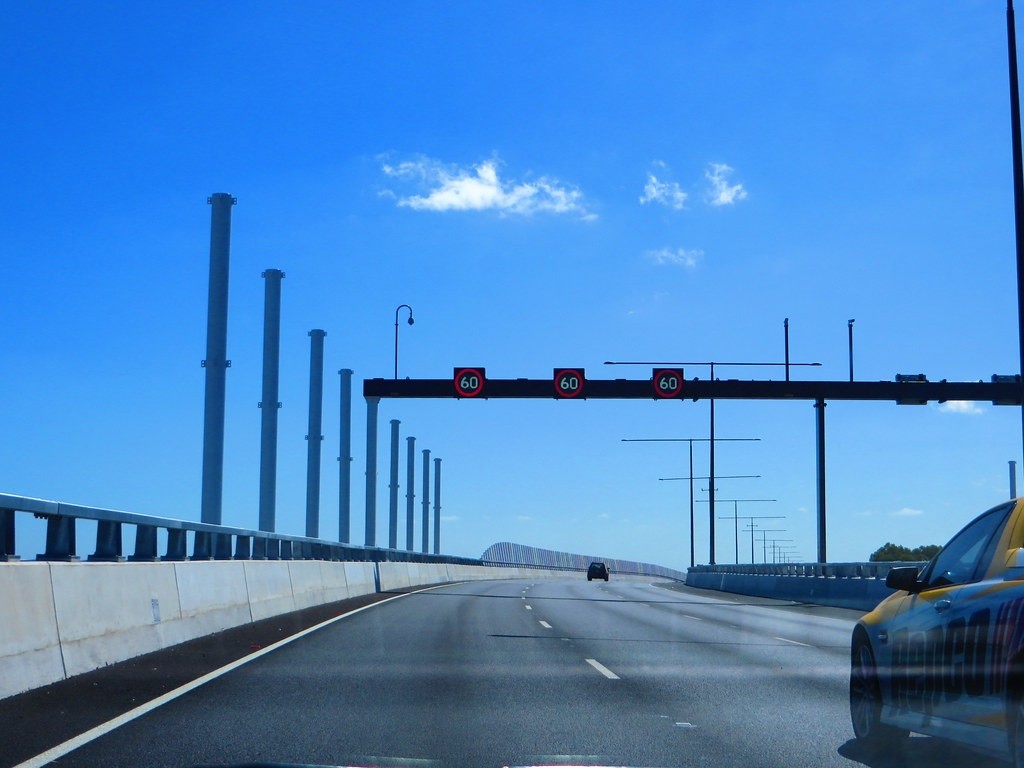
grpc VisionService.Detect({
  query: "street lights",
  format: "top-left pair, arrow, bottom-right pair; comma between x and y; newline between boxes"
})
604,361 -> 821,565
700,497 -> 805,564
621,438 -> 762,566
658,475 -> 760,565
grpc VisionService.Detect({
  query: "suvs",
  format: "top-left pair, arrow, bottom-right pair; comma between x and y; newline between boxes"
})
587,562 -> 611,582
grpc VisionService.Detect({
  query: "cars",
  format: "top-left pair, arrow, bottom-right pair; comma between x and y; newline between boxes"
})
847,496 -> 1024,768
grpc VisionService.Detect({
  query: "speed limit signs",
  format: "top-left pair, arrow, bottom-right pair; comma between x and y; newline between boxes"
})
553,368 -> 586,399
652,367 -> 685,400
454,367 -> 488,398
